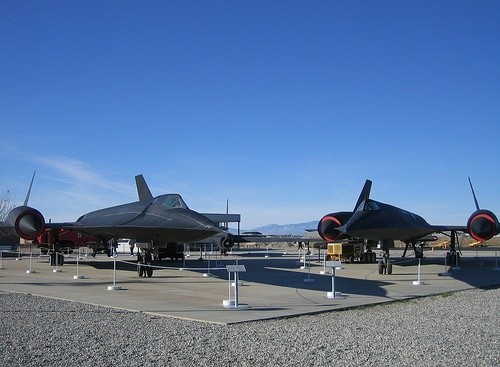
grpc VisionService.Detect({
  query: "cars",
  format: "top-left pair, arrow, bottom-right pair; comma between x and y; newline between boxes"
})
241,231 -> 262,236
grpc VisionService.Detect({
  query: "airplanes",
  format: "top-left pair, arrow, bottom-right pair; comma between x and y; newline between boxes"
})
0,171 -> 237,278
304,176 -> 500,274
